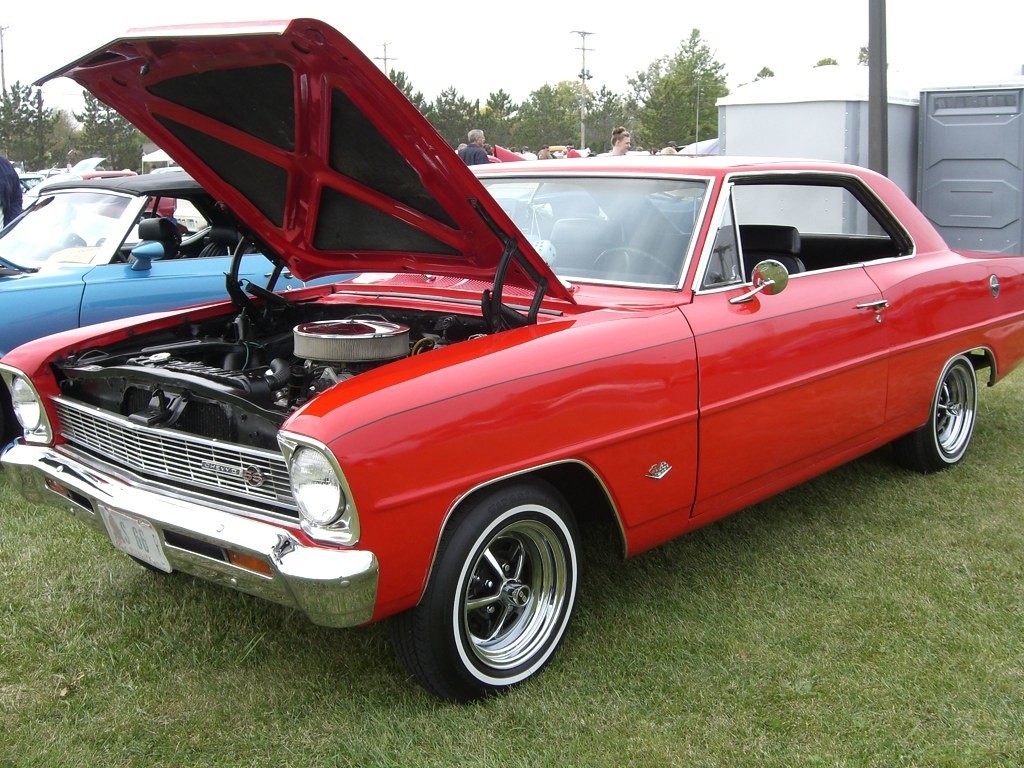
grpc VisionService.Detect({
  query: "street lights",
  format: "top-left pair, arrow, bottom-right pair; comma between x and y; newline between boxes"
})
570,30 -> 596,149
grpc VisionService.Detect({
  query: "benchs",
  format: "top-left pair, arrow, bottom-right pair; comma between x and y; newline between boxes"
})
639,232 -> 894,272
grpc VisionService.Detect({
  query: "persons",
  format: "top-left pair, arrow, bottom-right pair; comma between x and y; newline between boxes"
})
457,126 -> 679,167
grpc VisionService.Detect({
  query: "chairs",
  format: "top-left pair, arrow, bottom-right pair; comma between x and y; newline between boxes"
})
127,217 -> 181,263
197,225 -> 242,257
548,218 -> 624,269
715,225 -> 806,283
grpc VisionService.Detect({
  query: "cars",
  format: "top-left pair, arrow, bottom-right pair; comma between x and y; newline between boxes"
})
1,18 -> 1024,703
1,157 -> 360,448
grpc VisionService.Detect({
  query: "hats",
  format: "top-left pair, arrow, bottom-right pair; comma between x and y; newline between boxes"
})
661,147 -> 677,154
523,146 -> 528,150
668,141 -> 677,146
